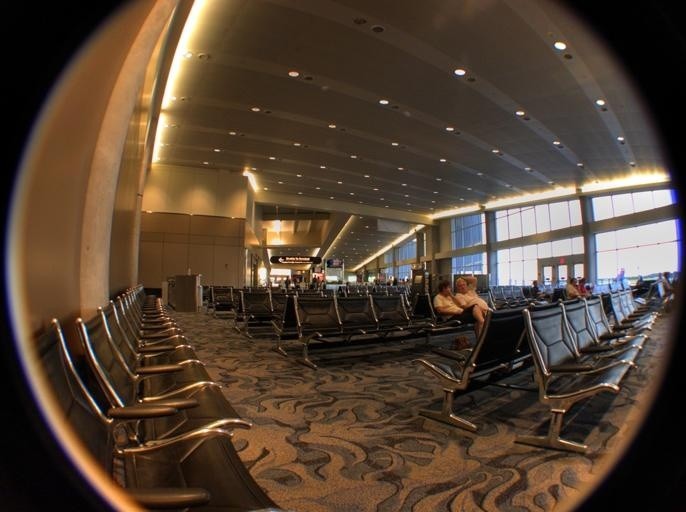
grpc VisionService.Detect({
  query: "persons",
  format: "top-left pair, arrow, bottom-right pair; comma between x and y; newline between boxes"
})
433,280 -> 484,340
375,274 -> 410,286
614,268 -> 680,314
532,280 -> 552,305
284,272 -> 327,290
566,276 -> 595,301
454,276 -> 493,320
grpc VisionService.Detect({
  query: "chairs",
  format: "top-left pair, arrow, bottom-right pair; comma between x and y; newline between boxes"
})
27,284 -> 285,508
202,283 -> 661,458
